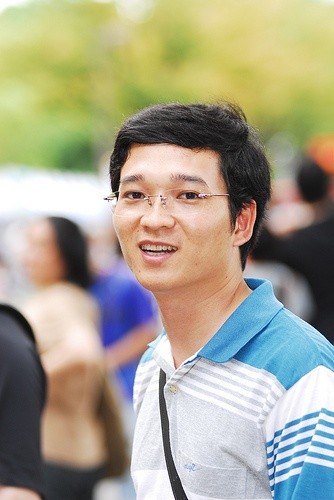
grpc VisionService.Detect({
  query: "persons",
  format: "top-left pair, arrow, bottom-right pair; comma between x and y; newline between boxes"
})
104,101 -> 334,500
0,297 -> 49,499
23,133 -> 334,500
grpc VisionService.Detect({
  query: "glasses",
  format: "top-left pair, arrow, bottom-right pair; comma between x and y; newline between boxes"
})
104,188 -> 230,217
